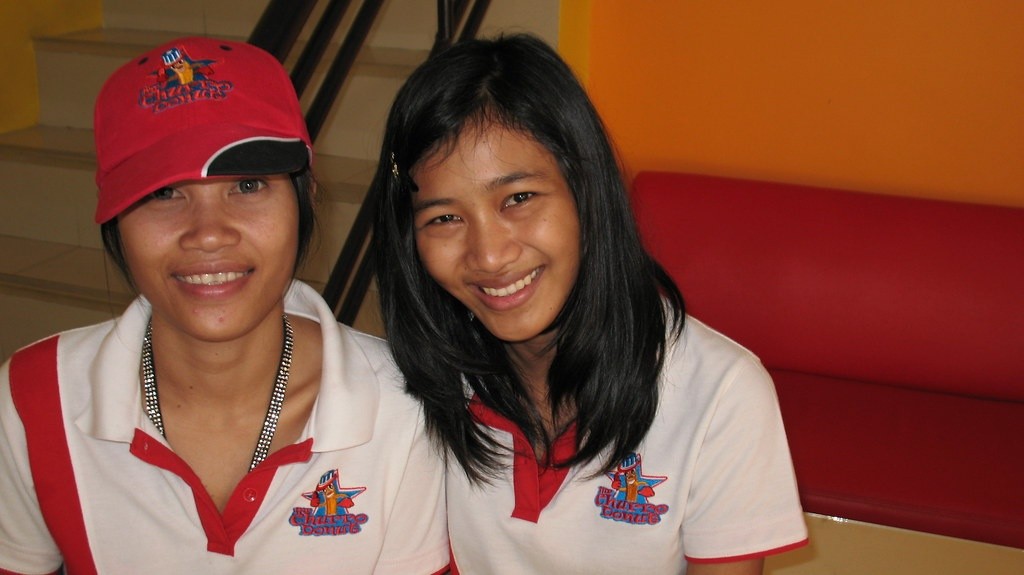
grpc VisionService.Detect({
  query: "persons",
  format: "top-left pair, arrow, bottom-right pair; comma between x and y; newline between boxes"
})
0,35 -> 449,575
369,31 -> 811,575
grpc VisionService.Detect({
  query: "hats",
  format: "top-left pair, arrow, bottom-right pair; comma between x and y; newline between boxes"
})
94,38 -> 314,225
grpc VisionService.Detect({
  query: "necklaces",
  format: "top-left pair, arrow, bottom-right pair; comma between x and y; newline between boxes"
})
142,313 -> 293,471
